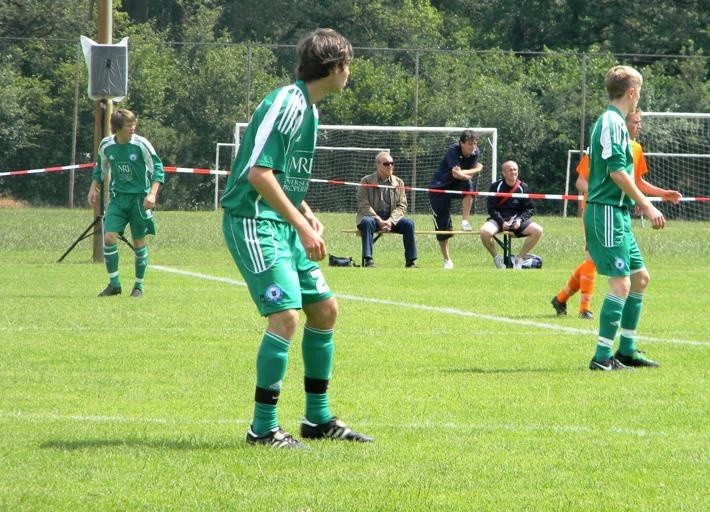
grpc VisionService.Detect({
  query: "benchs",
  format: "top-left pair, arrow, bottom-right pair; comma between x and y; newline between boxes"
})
341,229 -> 511,267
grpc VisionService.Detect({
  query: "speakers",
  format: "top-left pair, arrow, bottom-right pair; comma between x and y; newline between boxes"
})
88,40 -> 129,101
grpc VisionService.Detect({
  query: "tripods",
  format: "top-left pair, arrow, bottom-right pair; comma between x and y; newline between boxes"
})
51,103 -> 139,264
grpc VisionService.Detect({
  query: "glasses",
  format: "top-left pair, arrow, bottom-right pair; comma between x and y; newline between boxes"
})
383,162 -> 394,166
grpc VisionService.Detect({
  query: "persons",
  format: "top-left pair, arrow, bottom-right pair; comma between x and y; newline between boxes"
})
87,109 -> 165,299
355,152 -> 421,270
582,64 -> 666,373
480,160 -> 543,270
550,101 -> 682,321
427,130 -> 484,270
218,28 -> 374,452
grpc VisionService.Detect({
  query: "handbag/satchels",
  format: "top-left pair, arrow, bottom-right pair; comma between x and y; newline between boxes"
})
510,254 -> 542,268
329,254 -> 360,267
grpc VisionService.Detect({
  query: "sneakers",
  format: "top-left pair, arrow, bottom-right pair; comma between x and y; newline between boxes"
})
461,220 -> 472,231
511,256 -> 522,269
444,260 -> 453,269
246,424 -> 306,451
366,261 -> 375,268
589,356 -> 632,371
615,350 -> 660,367
130,288 -> 143,298
301,416 -> 373,443
493,255 -> 506,269
578,311 -> 594,320
406,262 -> 417,268
97,285 -> 121,297
551,296 -> 567,319
